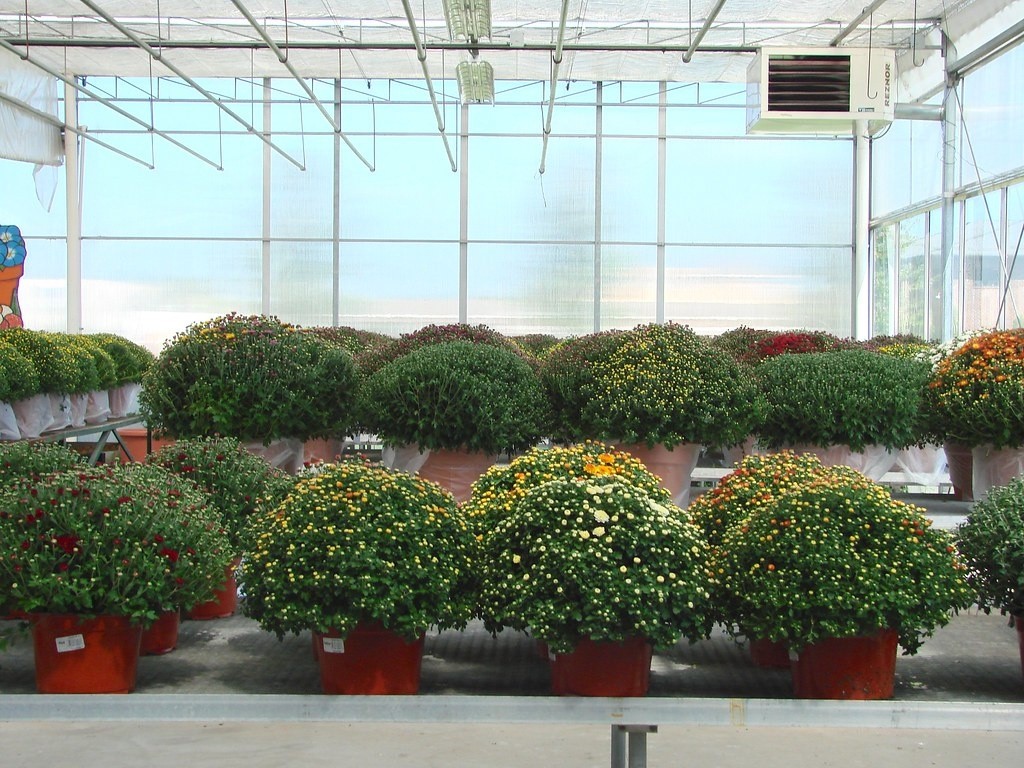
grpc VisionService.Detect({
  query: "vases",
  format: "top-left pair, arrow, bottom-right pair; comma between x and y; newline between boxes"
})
14,393 -> 41,438
179,556 -> 242,619
41,392 -> 67,432
0,398 -> 22,441
0,609 -> 30,620
69,392 -> 88,429
127,383 -> 142,413
789,624 -> 897,700
109,385 -> 127,419
87,388 -> 110,424
134,612 -> 181,657
314,619 -> 424,695
749,638 -> 790,670
113,426 -> 1024,509
546,632 -> 654,697
29,610 -> 143,693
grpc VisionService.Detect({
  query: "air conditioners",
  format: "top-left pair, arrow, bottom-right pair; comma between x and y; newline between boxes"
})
745,47 -> 897,136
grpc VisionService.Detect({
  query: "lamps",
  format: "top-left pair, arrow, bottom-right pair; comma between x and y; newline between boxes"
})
455,59 -> 496,108
441,0 -> 493,45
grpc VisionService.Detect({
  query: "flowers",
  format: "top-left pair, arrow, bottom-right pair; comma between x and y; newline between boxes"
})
707,465 -> 979,657
470,437 -> 673,544
0,327 -> 81,394
85,333 -> 142,385
65,332 -> 121,390
117,337 -> 155,383
60,339 -> 101,394
137,317 -> 1024,453
0,464 -> 220,631
238,453 -> 391,549
690,447 -> 832,551
481,474 -> 717,654
954,474 -> 1024,627
0,438 -> 94,611
234,451 -> 483,643
146,431 -> 293,550
0,338 -> 41,402
